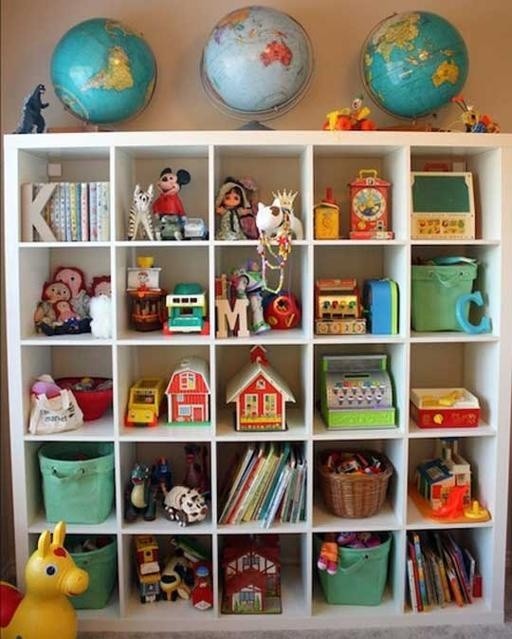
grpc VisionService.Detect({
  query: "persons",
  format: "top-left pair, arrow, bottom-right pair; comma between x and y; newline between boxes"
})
53,298 -> 81,332
180,452 -> 208,496
216,175 -> 251,241
150,457 -> 174,505
88,276 -> 110,298
34,281 -> 79,335
54,265 -> 93,333
233,258 -> 271,335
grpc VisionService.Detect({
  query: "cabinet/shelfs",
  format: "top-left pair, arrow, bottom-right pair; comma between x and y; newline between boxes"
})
2,132 -> 511,633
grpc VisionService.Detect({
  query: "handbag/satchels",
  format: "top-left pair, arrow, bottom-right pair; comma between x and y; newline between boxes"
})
28,374 -> 83,434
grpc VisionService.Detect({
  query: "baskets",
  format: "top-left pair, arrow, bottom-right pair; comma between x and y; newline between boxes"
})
314,449 -> 394,519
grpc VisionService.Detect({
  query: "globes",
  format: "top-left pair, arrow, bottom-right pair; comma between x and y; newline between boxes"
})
46,18 -> 158,132
200,5 -> 316,132
358,10 -> 469,133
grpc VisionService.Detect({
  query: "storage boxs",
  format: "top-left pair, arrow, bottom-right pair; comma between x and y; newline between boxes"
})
63,535 -> 116,609
412,263 -> 477,332
313,533 -> 392,605
37,442 -> 114,524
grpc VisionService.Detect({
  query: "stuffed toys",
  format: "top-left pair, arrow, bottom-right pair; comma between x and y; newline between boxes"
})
126,464 -> 156,523
127,181 -> 157,240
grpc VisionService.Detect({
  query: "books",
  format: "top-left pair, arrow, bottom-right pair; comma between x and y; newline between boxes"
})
407,531 -> 483,614
27,180 -> 109,242
215,443 -> 306,532
